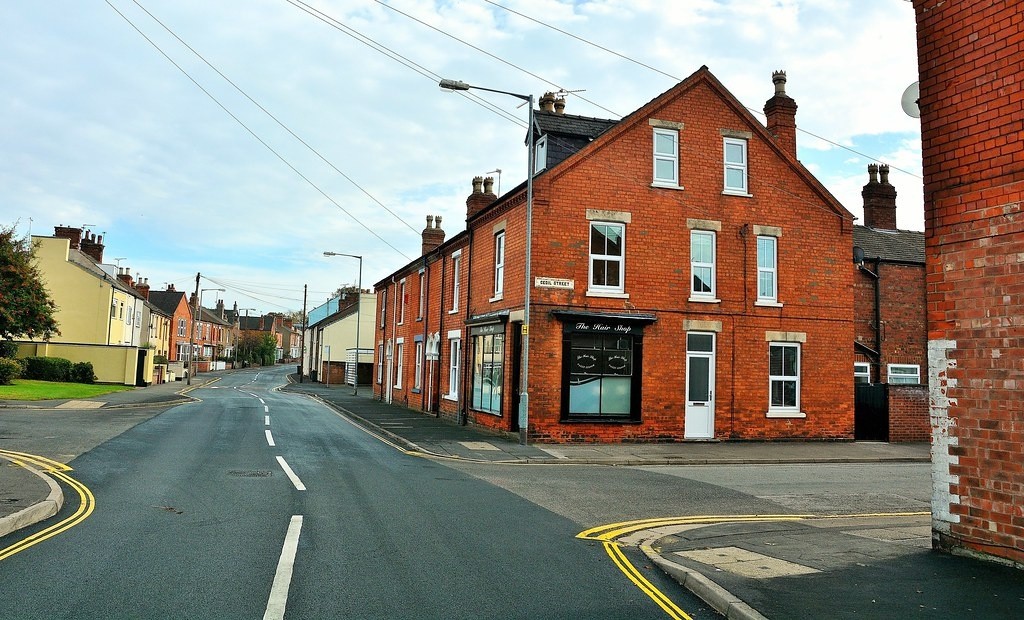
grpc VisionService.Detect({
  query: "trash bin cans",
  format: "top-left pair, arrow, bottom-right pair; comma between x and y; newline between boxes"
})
296,365 -> 303,375
310,370 -> 317,382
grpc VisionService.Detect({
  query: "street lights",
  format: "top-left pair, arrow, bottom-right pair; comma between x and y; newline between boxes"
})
195,288 -> 226,378
235,307 -> 256,368
323,251 -> 362,396
439,76 -> 535,446
